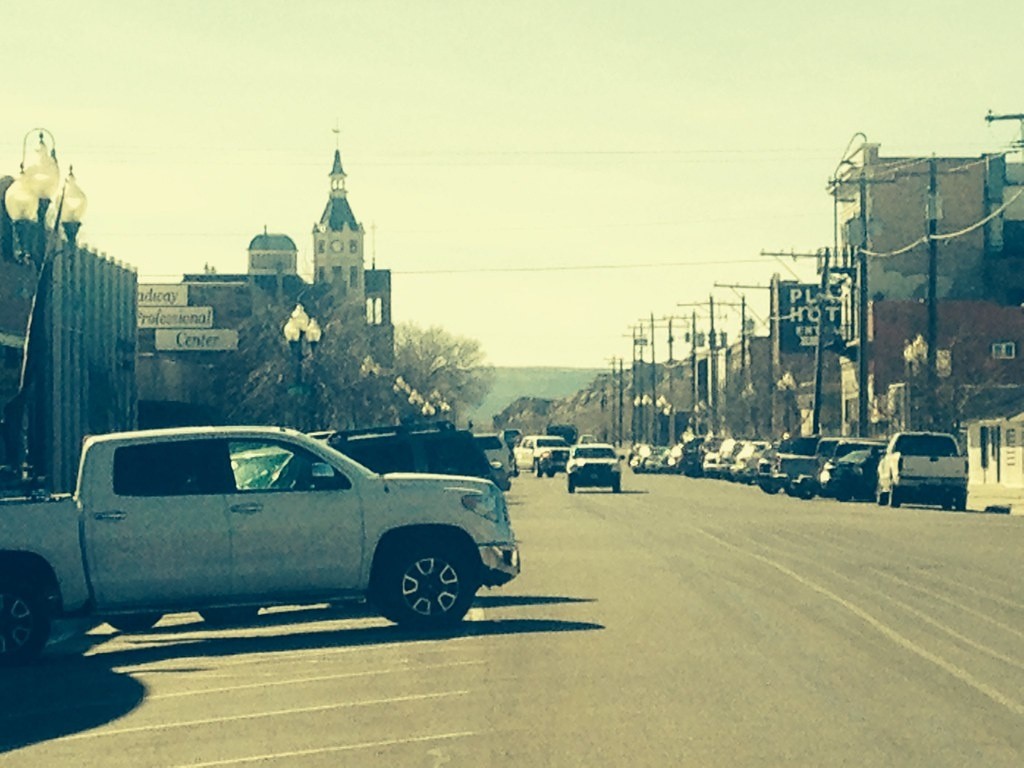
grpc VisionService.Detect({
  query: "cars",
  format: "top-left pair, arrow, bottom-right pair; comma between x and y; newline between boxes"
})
626,437 -> 889,499
470,436 -> 507,469
827,440 -> 883,502
510,433 -> 574,476
567,444 -> 624,491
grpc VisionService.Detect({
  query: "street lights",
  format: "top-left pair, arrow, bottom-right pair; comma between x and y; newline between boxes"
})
358,355 -> 382,428
905,333 -> 926,434
283,305 -> 321,427
633,394 -> 710,449
390,374 -> 409,428
405,389 -> 423,424
777,371 -> 798,438
6,125 -> 86,418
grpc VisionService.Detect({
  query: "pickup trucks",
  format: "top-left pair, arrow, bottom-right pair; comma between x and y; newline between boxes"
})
1,425 -> 520,670
878,428 -> 974,512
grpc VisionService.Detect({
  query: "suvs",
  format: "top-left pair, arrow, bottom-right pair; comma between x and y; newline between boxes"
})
219,428 -> 508,496
